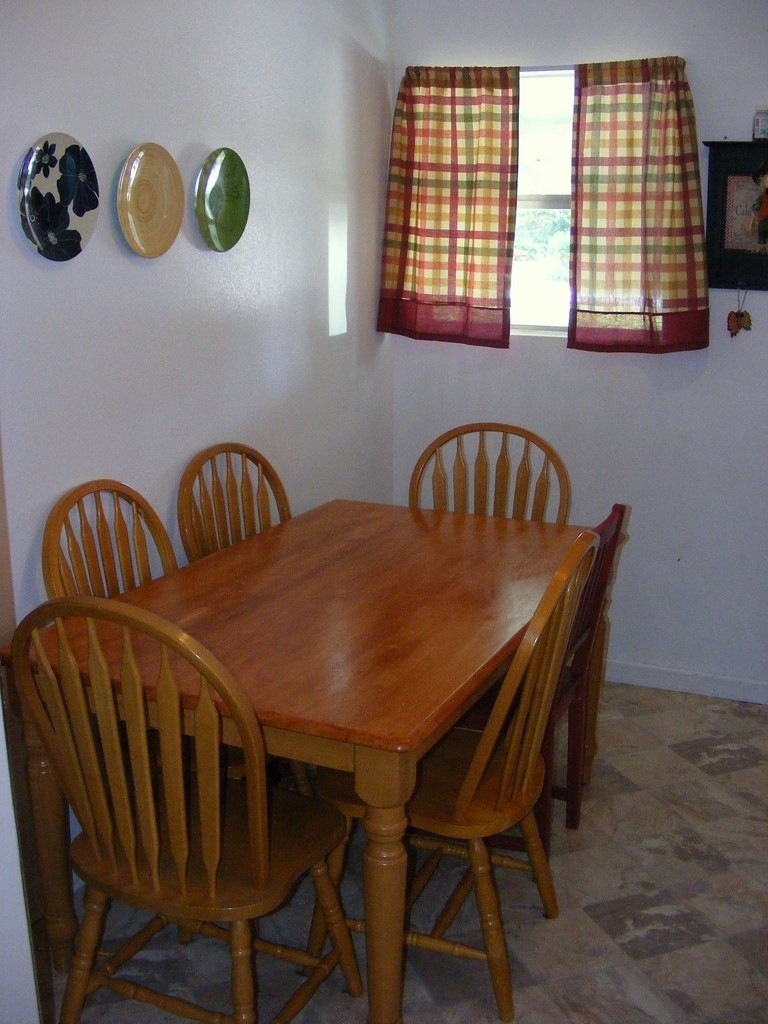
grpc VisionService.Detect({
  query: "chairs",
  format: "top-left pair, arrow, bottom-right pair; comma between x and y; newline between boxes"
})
176,442 -> 292,563
10,596 -> 363,1024
306,529 -> 600,1023
409,423 -> 568,523
42,479 -> 178,600
452,503 -> 633,864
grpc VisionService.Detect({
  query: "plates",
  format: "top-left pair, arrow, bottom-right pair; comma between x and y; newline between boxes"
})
117,143 -> 184,258
195,147 -> 250,253
16,132 -> 99,262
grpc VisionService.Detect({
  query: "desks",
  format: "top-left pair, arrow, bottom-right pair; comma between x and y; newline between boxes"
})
0,499 -> 598,1024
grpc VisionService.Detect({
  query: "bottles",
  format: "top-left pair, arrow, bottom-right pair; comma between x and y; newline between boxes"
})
753,104 -> 768,142
